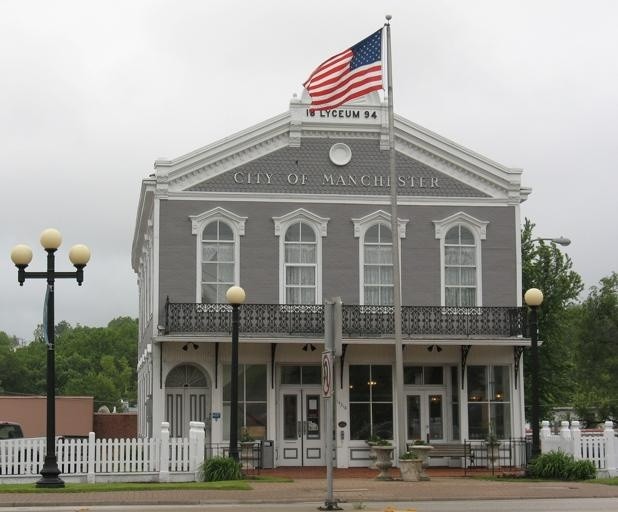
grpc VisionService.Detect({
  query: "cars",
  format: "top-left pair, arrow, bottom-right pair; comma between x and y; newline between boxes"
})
576,418 -> 617,436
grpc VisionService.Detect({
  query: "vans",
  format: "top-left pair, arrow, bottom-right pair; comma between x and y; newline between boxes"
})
57,433 -> 89,473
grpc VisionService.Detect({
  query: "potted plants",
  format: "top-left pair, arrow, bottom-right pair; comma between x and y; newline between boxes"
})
370,440 -> 394,482
485,432 -> 500,469
407,437 -> 434,481
399,451 -> 423,483
241,428 -> 256,470
366,432 -> 385,468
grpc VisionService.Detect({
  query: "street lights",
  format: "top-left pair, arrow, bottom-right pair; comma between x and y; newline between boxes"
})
522,287 -> 544,464
224,285 -> 246,465
522,235 -> 570,248
9,228 -> 89,490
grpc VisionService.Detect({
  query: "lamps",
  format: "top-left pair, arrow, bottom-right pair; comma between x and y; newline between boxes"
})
301,343 -> 317,352
182,342 -> 199,353
521,234 -> 571,251
426,345 -> 443,353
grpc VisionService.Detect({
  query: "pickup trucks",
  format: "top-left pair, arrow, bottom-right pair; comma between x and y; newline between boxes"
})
0,421 -> 39,475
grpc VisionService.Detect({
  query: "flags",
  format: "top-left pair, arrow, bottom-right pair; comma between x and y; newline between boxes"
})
302,22 -> 388,113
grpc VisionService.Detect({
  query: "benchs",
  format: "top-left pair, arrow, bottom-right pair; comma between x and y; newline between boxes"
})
406,442 -> 472,477
